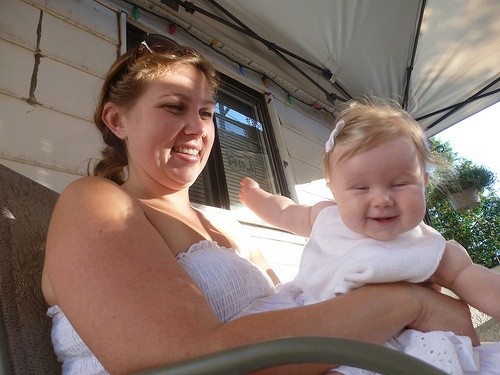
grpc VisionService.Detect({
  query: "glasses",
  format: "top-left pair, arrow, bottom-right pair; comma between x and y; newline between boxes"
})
129,32 -> 201,72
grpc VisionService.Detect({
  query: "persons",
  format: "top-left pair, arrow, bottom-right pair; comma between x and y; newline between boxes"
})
39,35 -> 485,375
235,95 -> 498,375
